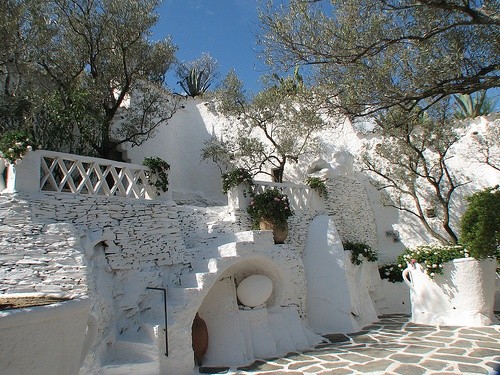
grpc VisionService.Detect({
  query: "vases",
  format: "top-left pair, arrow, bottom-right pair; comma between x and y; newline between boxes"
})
257,214 -> 289,244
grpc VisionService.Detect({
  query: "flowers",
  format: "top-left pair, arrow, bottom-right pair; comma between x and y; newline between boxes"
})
221,168 -> 254,196
246,186 -> 295,234
